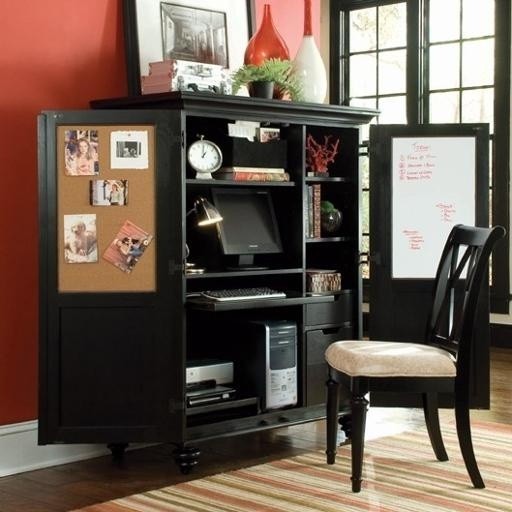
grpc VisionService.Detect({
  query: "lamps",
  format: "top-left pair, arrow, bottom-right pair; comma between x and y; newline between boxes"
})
186,198 -> 222,274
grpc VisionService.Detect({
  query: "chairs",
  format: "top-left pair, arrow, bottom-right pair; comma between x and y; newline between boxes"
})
325,223 -> 505,493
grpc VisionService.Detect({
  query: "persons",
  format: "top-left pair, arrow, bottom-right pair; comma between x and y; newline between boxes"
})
69,139 -> 97,175
116,239 -> 144,268
107,184 -> 120,205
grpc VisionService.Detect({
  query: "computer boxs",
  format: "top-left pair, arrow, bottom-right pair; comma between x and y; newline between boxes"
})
244,322 -> 300,410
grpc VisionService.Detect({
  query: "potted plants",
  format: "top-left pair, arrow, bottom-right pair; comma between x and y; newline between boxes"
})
321,200 -> 342,237
230,58 -> 306,101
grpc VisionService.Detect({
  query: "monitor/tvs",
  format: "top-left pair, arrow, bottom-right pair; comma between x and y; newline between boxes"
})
213,187 -> 283,269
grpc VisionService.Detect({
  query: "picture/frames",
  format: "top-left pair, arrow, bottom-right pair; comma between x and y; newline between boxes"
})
121,0 -> 257,97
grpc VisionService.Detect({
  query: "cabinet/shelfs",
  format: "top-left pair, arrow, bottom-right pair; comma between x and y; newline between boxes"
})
304,123 -> 359,408
182,109 -> 305,429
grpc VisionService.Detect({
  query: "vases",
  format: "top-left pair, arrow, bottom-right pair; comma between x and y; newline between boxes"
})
245,5 -> 291,100
292,0 -> 328,104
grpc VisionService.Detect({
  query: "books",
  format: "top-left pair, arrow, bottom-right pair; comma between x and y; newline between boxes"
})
308,184 -> 321,237
215,167 -> 290,180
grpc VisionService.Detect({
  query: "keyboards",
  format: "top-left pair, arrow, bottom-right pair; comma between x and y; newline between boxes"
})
201,286 -> 287,300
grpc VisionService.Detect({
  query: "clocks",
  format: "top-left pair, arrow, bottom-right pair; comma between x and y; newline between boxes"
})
187,134 -> 223,179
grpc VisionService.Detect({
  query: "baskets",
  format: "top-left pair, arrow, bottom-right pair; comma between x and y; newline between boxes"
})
147,59 -> 228,80
139,75 -> 233,97
